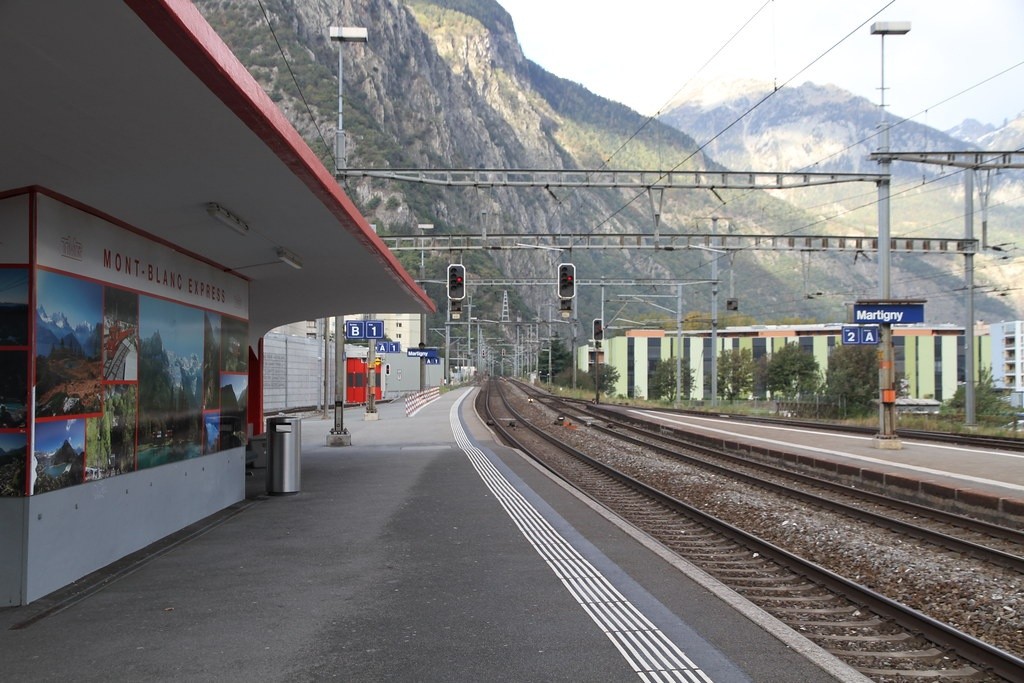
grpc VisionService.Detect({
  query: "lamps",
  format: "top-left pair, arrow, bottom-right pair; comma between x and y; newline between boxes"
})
207,202 -> 248,236
276,248 -> 305,269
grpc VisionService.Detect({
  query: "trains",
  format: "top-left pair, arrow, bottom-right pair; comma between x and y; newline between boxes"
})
106,326 -> 135,359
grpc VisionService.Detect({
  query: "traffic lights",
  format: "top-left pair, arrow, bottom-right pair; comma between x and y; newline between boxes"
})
446,264 -> 466,300
558,263 -> 576,299
592,318 -> 604,342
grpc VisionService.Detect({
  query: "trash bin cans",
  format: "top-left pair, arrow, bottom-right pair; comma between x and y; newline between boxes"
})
265,416 -> 302,493
